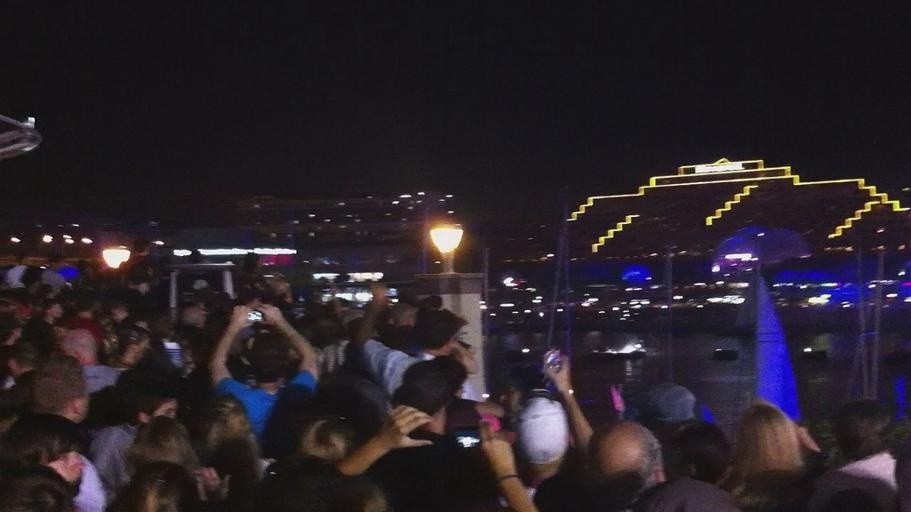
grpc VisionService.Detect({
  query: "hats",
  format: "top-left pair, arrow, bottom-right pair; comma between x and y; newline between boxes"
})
403,354 -> 467,410
626,379 -> 697,422
521,397 -> 569,464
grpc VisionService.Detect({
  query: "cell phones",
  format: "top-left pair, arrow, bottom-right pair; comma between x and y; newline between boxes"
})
543,345 -> 564,376
246,311 -> 265,322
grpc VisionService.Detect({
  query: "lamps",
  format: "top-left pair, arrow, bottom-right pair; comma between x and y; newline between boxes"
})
428,220 -> 466,273
102,244 -> 133,270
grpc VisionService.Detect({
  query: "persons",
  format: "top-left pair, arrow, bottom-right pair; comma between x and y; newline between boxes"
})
480,355 -> 910,511
1,233 -> 517,512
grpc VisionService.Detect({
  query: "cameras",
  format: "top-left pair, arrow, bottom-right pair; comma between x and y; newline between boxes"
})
451,427 -> 483,452
